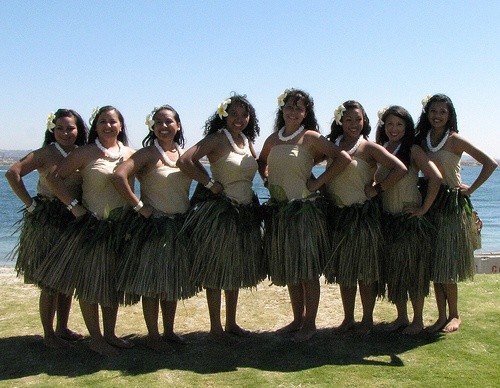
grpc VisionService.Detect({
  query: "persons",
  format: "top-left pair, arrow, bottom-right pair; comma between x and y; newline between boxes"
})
314,99 -> 407,341
414,94 -> 498,333
376,105 -> 443,335
175,96 -> 260,348
256,88 -> 352,344
113,105 -> 210,355
5,108 -> 89,351
221,126 -> 249,155
46,105 -> 141,359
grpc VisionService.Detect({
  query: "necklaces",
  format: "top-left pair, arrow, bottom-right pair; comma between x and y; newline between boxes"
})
426,128 -> 450,152
278,124 -> 306,141
50,141 -> 69,158
94,138 -> 123,159
154,139 -> 183,167
383,140 -> 402,156
336,134 -> 364,156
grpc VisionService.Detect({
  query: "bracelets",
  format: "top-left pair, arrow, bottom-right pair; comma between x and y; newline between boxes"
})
374,184 -> 385,194
67,198 -> 79,211
204,177 -> 215,189
262,178 -> 268,183
27,199 -> 38,212
133,200 -> 145,212
